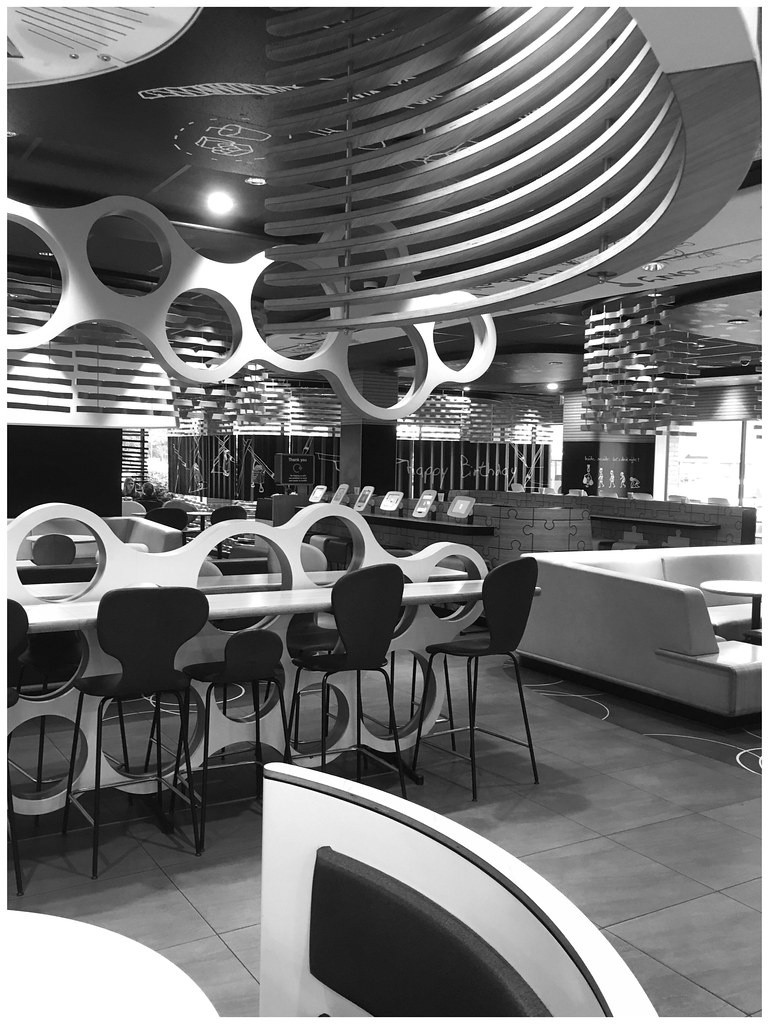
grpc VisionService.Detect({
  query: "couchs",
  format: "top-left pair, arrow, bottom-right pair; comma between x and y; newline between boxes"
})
521,543 -> 761,732
7,516 -> 182,564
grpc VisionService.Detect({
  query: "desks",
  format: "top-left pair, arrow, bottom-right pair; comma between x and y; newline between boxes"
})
131,511 -> 213,536
700,580 -> 761,647
8,567 -> 541,821
294,506 -> 495,536
590,515 -> 721,530
25,533 -> 97,544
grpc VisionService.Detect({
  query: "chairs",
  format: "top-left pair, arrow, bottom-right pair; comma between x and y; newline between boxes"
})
259,761 -> 656,1017
122,499 -> 247,559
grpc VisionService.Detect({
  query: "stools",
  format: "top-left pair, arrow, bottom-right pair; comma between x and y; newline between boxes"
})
304,534 -> 465,614
413,557 -> 538,802
7,599 -> 29,896
59,586 -> 199,878
283,562 -> 407,806
167,628 -> 293,852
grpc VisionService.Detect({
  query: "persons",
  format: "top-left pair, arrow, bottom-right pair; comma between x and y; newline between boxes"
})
122,478 -> 140,499
134,483 -> 159,502
